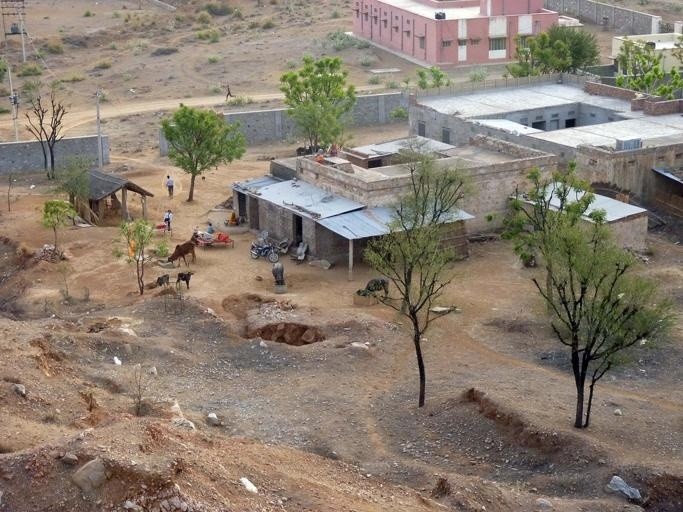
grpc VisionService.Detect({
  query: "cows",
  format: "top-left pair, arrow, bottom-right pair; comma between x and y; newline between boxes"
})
357,278 -> 389,304
176,271 -> 194,290
156,274 -> 169,288
168,240 -> 197,267
272,262 -> 284,285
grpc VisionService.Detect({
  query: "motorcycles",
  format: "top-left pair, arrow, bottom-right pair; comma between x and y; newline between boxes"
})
249,239 -> 279,262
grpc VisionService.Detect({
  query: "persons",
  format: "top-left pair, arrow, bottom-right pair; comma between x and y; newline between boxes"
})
164,175 -> 173,199
327,142 -> 340,165
193,225 -> 199,237
224,85 -> 235,103
162,209 -> 173,231
312,148 -> 323,180
206,222 -> 214,234
